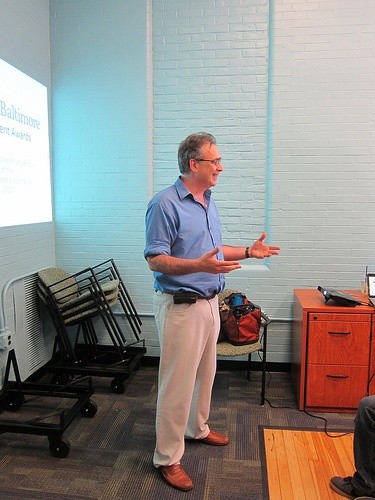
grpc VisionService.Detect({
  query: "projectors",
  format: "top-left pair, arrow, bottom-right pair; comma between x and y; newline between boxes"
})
317,286 -> 362,306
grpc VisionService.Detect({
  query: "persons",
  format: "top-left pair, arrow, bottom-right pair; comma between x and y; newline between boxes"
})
329,395 -> 375,500
144,131 -> 281,491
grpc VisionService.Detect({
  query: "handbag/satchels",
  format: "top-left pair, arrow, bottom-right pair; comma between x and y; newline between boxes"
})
220,293 -> 261,346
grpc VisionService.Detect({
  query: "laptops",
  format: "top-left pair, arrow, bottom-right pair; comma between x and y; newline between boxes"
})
367,274 -> 375,306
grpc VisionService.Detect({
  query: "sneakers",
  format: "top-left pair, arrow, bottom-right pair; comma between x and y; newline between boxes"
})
330,476 -> 361,498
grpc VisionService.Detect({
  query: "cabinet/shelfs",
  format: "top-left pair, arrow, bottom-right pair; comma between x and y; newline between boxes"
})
293,288 -> 375,413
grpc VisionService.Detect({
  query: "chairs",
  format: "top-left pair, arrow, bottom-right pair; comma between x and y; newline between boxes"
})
36,258 -> 142,364
216,290 -> 271,407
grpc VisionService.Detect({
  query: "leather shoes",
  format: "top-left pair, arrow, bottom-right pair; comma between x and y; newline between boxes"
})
184,430 -> 230,446
159,465 -> 194,491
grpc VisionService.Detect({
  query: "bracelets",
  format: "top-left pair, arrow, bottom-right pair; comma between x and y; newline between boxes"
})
245,247 -> 250,259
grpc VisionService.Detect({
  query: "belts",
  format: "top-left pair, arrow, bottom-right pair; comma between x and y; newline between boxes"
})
165,292 -> 216,300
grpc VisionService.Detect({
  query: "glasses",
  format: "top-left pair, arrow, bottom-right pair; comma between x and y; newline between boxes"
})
187,158 -> 221,166
